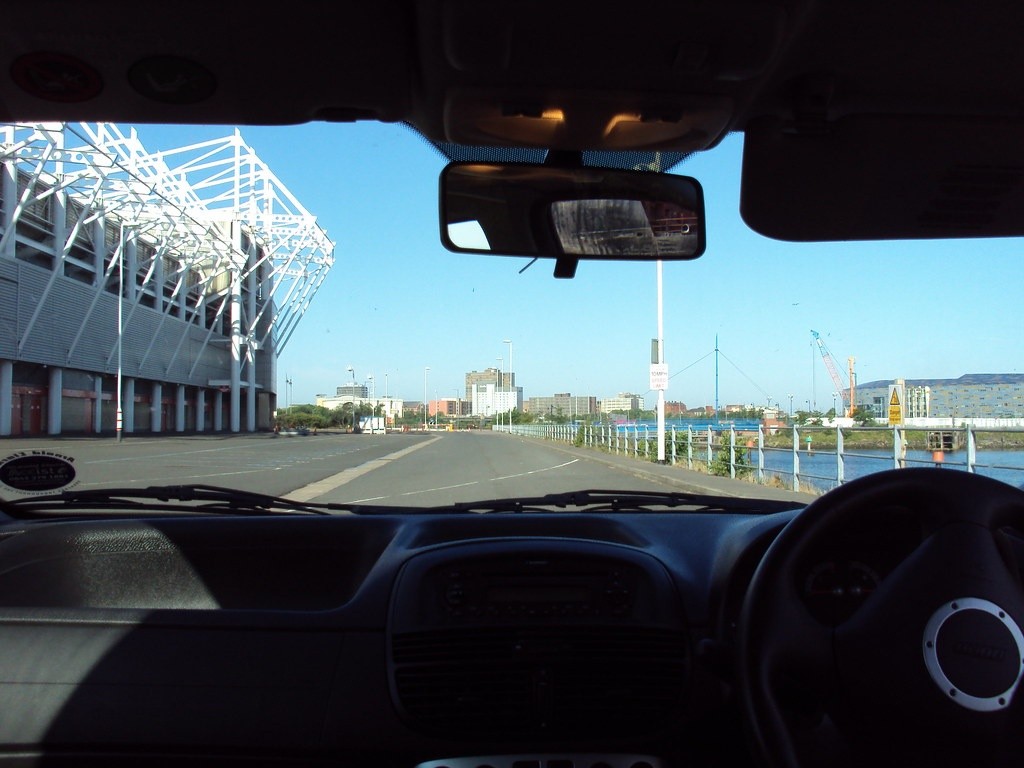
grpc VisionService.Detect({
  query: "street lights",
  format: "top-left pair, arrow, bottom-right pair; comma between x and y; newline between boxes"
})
492,367 -> 498,431
386,372 -> 388,425
496,357 -> 504,432
425,367 -> 430,430
368,375 -> 374,417
788,394 -> 793,416
347,367 -> 355,425
832,392 -> 838,417
503,340 -> 512,433
751,402 -> 754,409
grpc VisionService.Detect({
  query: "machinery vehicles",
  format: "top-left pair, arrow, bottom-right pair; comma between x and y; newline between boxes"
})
809,330 -> 858,417
